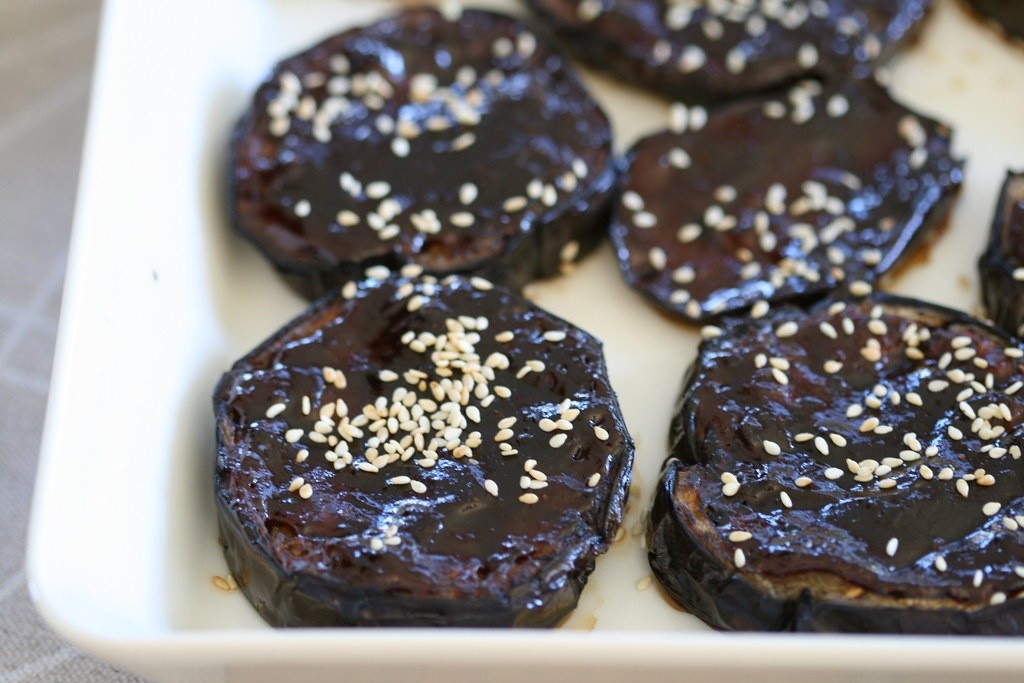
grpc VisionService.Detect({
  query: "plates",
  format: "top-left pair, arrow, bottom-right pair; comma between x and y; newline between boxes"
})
26,1 -> 1023,681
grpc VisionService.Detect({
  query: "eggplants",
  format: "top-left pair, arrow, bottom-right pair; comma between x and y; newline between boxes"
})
210,0 -> 1024,636
